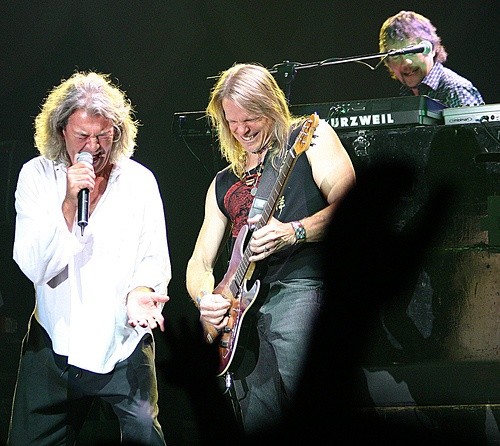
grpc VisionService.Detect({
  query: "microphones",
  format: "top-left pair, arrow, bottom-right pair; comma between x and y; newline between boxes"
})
387,40 -> 433,55
76,151 -> 94,227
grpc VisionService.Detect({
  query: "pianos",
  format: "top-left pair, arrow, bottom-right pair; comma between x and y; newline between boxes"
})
174,95 -> 446,136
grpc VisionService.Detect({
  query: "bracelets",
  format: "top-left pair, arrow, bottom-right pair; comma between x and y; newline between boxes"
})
290,220 -> 306,248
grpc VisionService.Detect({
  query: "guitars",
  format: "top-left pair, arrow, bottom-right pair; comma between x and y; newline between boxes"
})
212,111 -> 320,385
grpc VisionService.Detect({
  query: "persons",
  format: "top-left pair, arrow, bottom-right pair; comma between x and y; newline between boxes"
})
1,71 -> 175,446
184,64 -> 358,437
379,10 -> 486,110
